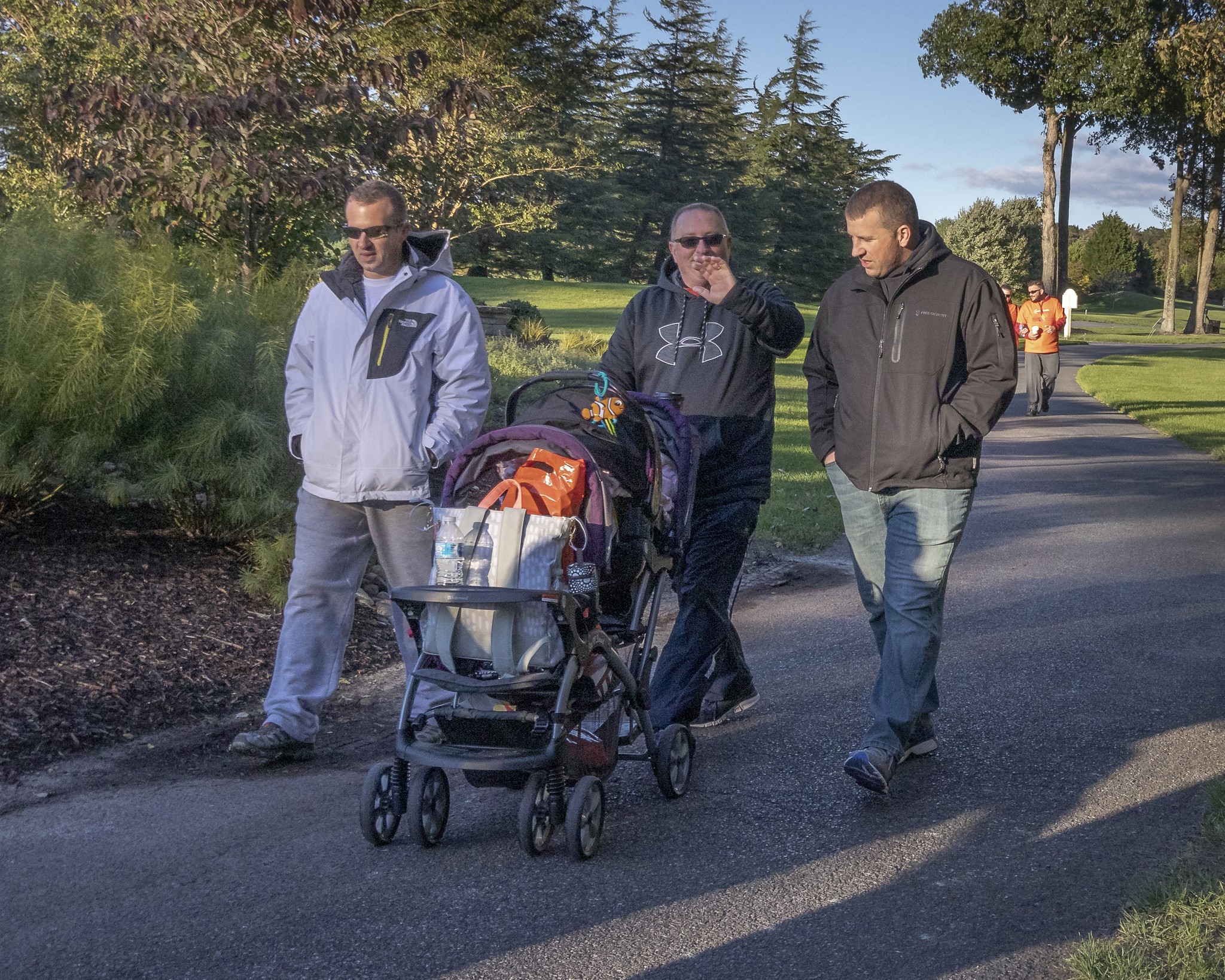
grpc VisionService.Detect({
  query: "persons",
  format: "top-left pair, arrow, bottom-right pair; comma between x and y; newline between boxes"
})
594,200 -> 809,735
1015,278 -> 1067,418
801,178 -> 1021,797
999,283 -> 1029,347
225,178 -> 494,772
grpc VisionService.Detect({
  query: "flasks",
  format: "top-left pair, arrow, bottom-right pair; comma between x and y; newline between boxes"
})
564,562 -> 599,592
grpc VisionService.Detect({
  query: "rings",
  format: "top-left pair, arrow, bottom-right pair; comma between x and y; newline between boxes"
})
715,266 -> 721,271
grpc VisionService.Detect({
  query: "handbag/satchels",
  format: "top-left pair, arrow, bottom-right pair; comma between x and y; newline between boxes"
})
419,505 -> 574,674
503,449 -> 586,576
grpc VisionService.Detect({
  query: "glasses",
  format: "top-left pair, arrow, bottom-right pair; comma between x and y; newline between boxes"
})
1028,289 -> 1040,295
339,224 -> 396,237
675,234 -> 727,248
1003,293 -> 1012,298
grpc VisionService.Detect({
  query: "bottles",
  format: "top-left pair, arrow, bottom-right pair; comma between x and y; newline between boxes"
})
434,516 -> 465,587
1019,322 -> 1025,337
463,521 -> 494,587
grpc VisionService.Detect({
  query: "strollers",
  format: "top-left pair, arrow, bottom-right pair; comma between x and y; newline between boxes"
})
357,370 -> 695,859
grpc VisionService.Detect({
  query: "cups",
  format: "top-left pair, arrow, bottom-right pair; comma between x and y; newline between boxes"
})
1032,326 -> 1039,334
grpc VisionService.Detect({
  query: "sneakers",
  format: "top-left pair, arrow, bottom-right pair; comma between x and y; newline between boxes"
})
1026,410 -> 1036,416
692,684 -> 763,732
844,739 -> 895,792
899,729 -> 937,764
233,724 -> 315,757
1042,404 -> 1049,412
